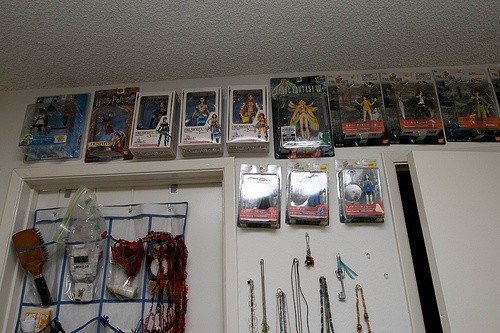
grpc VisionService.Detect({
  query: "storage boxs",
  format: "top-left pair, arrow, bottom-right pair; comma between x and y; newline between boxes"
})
225,84 -> 270,153
129,90 -> 181,159
178,87 -> 224,157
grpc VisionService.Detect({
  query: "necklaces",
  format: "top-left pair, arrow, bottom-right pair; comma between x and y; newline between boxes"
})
247,234 -> 373,333
